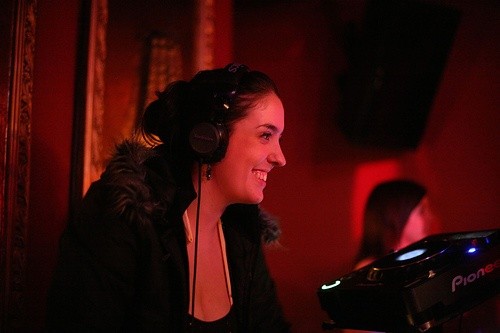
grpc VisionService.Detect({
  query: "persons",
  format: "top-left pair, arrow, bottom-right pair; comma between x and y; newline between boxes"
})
347,178 -> 435,333
41,63 -> 296,333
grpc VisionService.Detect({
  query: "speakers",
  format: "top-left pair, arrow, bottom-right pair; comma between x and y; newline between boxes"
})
333,0 -> 462,149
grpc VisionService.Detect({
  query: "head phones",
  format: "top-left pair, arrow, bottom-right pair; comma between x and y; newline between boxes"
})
186,63 -> 249,163
